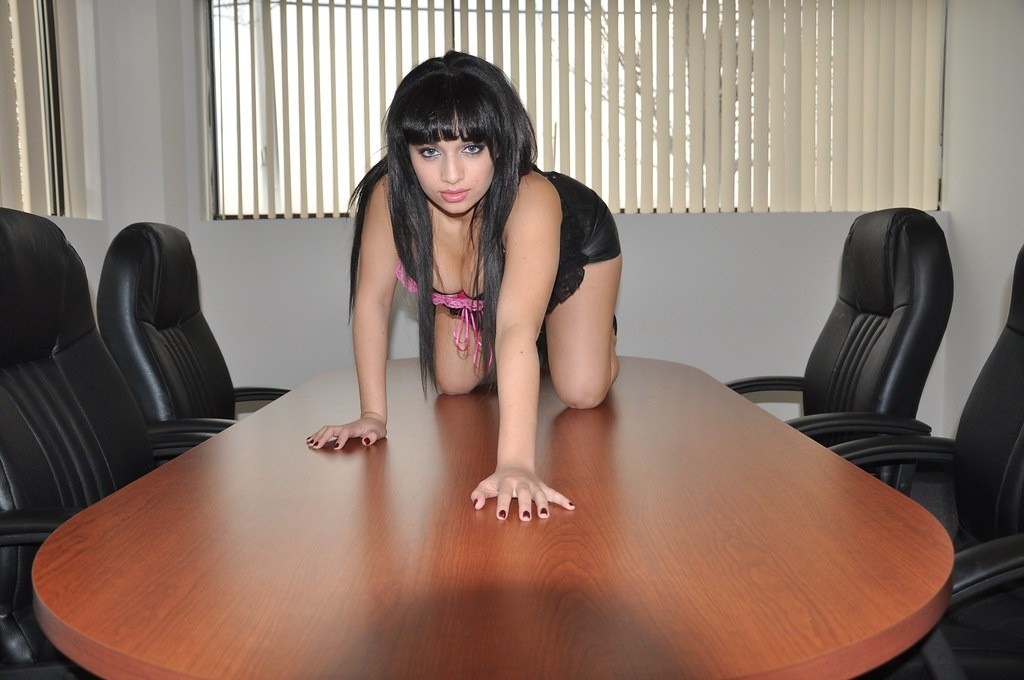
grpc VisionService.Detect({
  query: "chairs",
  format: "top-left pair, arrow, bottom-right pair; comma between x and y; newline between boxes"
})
0,207 -> 219,680
97,223 -> 291,431
832,245 -> 1024,678
727,209 -> 954,445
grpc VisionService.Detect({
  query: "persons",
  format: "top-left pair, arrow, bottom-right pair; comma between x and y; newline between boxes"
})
307,49 -> 623,520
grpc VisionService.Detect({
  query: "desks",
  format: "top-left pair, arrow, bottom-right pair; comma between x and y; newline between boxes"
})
33,354 -> 955,679
